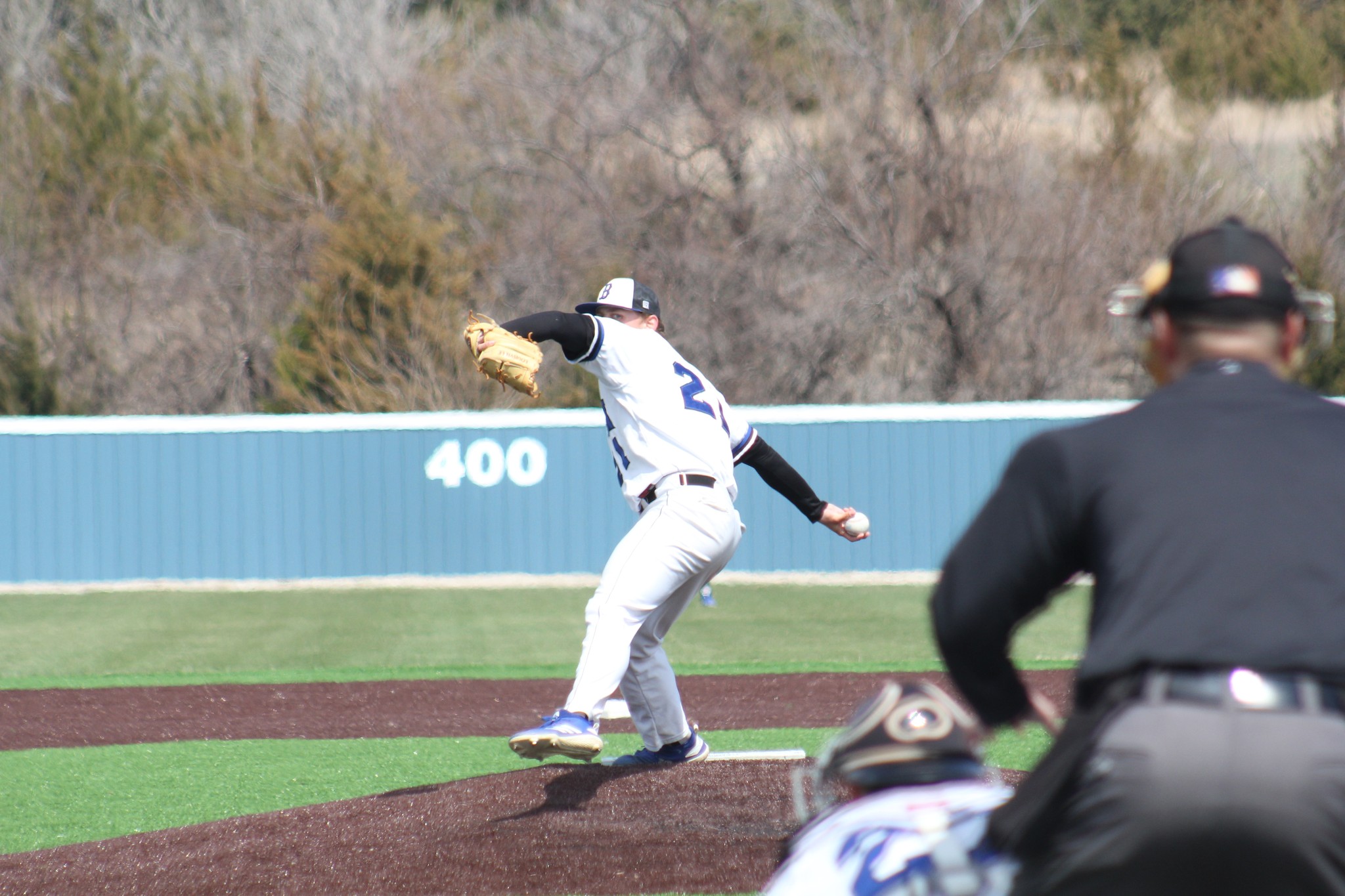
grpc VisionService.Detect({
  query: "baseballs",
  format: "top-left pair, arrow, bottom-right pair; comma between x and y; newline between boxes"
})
844,512 -> 869,537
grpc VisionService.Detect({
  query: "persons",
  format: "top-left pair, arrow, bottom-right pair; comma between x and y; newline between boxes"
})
760,678 -> 1025,896
929,214 -> 1345,896
464,278 -> 871,766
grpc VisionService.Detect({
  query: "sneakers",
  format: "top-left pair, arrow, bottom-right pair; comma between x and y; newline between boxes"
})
508,708 -> 606,762
609,727 -> 707,770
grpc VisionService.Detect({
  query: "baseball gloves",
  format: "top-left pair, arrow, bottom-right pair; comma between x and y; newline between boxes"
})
463,319 -> 546,398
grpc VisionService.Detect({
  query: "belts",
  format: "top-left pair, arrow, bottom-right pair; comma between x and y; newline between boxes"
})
637,474 -> 716,512
1073,673 -> 1345,714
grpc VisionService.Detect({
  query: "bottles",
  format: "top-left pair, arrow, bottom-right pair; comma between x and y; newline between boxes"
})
700,583 -> 714,606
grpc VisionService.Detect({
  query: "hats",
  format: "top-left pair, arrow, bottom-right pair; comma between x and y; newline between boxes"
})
574,278 -> 663,321
1124,213 -> 1302,316
815,678 -> 981,786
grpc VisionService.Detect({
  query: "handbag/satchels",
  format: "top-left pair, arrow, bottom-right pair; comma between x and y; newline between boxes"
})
986,705 -> 1119,855
